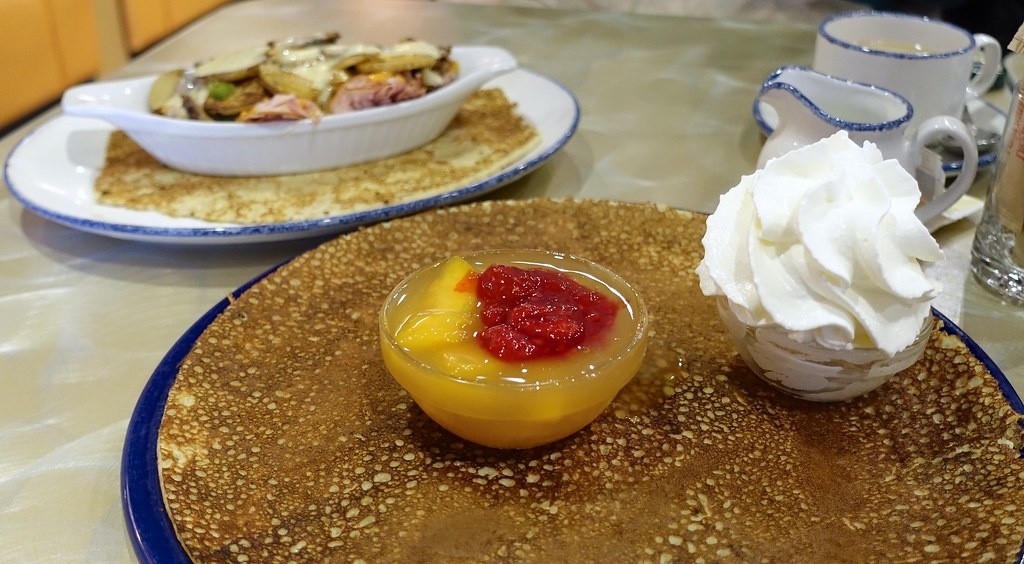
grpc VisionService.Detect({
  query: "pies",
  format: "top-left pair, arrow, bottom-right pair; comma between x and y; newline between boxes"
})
92,87 -> 542,223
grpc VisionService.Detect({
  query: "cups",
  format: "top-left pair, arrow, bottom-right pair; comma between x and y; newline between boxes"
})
814,9 -> 1001,133
970,78 -> 1024,306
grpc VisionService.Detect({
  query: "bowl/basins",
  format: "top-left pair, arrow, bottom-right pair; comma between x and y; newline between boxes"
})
710,294 -> 934,402
378,247 -> 648,449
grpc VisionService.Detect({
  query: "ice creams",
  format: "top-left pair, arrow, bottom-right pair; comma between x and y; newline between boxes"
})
695,131 -> 947,405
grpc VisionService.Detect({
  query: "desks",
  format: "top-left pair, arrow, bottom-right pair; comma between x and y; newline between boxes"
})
1,2 -> 1023,564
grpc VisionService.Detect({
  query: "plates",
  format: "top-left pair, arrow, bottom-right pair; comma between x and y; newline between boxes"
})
4,60 -> 580,243
59,39 -> 517,175
931,98 -> 1010,179
119,200 -> 1023,563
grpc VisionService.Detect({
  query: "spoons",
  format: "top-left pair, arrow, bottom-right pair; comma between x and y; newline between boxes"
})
940,102 -> 996,156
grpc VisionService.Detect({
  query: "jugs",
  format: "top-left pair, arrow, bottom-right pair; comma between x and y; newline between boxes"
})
752,64 -> 979,235
